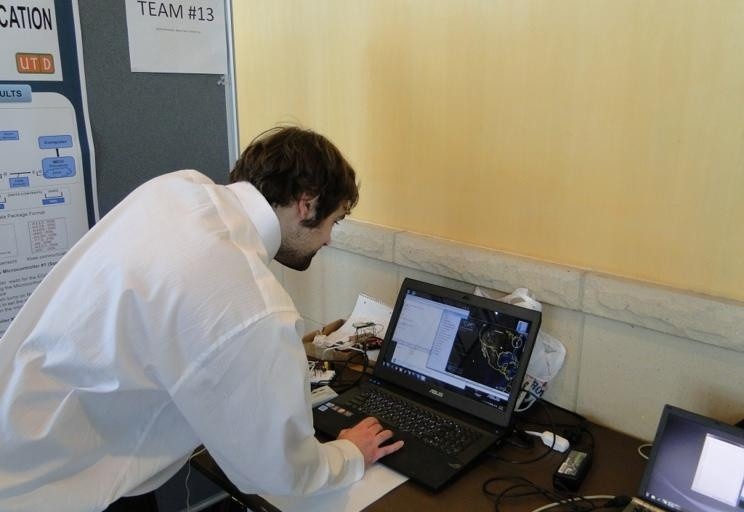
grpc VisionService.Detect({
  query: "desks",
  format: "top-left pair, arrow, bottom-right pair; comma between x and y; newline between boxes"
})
181,341 -> 653,512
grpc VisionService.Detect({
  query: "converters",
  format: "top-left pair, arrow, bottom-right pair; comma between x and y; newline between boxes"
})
552,448 -> 593,495
541,430 -> 570,453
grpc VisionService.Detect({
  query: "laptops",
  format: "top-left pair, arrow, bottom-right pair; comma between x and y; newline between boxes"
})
313,278 -> 542,495
623,405 -> 744,512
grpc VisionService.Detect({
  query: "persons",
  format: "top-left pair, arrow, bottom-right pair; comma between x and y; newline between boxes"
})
1,125 -> 403,511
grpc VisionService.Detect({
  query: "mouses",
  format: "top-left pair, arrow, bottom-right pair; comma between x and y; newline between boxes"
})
364,337 -> 383,350
